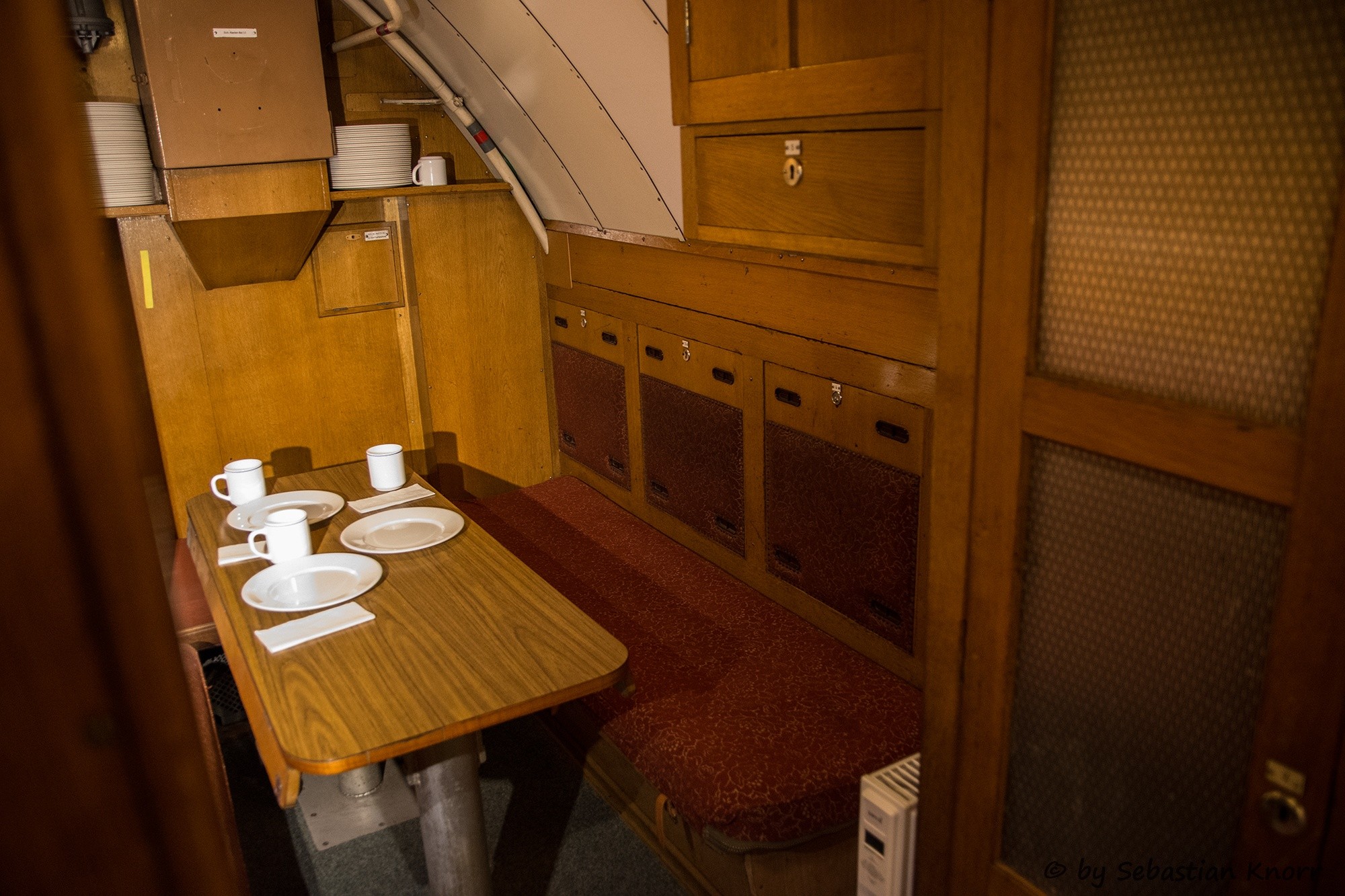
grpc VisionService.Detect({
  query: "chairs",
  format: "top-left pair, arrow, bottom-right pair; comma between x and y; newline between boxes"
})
166,534 -> 221,652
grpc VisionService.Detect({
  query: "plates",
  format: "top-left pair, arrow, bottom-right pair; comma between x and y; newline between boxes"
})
75,101 -> 156,209
327,123 -> 412,191
226,490 -> 345,532
240,552 -> 383,612
340,506 -> 465,555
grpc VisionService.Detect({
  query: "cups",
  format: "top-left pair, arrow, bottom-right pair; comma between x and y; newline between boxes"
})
412,156 -> 447,187
211,458 -> 267,507
366,443 -> 407,492
248,507 -> 314,564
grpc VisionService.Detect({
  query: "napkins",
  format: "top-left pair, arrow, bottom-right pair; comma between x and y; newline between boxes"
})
253,601 -> 376,654
347,483 -> 435,514
218,541 -> 267,566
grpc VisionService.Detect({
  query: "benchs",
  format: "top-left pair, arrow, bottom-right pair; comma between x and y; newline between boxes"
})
456,474 -> 926,896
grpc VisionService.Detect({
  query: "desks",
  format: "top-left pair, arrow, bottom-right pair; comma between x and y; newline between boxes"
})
183,455 -> 629,896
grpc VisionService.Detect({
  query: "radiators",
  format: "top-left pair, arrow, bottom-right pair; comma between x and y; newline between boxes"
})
857,752 -> 920,896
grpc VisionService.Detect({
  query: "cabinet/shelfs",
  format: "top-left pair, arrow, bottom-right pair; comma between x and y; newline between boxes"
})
548,302 -> 926,687
665,0 -> 942,255
